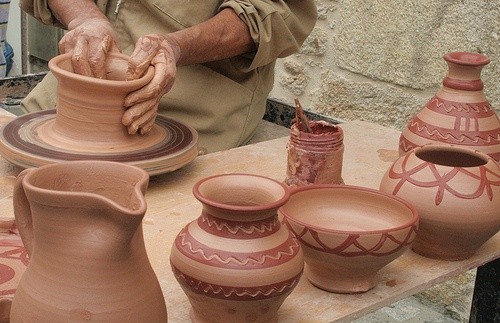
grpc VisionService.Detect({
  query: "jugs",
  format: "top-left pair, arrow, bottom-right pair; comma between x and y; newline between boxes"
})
9,160 -> 168,323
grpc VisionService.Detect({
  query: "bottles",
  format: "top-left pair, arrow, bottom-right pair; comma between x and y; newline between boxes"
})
380,143 -> 500,261
398,51 -> 500,165
285,121 -> 346,187
170,173 -> 304,323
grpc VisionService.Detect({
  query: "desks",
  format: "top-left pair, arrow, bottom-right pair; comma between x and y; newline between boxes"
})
1,106 -> 500,322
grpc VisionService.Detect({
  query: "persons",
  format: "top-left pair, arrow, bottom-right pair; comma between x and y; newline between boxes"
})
18,0 -> 318,158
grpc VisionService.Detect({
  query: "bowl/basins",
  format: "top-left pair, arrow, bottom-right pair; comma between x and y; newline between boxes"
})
278,184 -> 422,294
